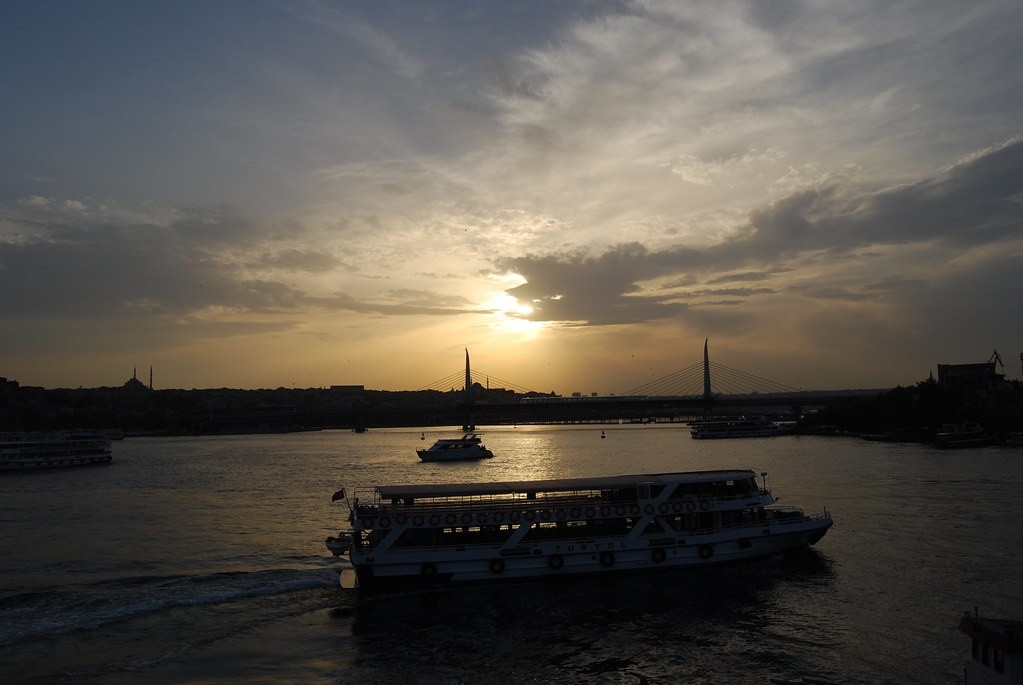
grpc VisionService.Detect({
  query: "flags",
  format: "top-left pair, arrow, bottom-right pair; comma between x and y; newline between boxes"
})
331,489 -> 345,502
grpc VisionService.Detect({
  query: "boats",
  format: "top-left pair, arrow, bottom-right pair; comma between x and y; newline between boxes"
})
1006,432 -> 1023,446
0,432 -> 112,472
935,424 -> 985,449
107,429 -> 126,440
690,421 -> 775,429
771,412 -> 799,427
415,434 -> 495,461
325,469 -> 835,597
692,425 -> 797,439
